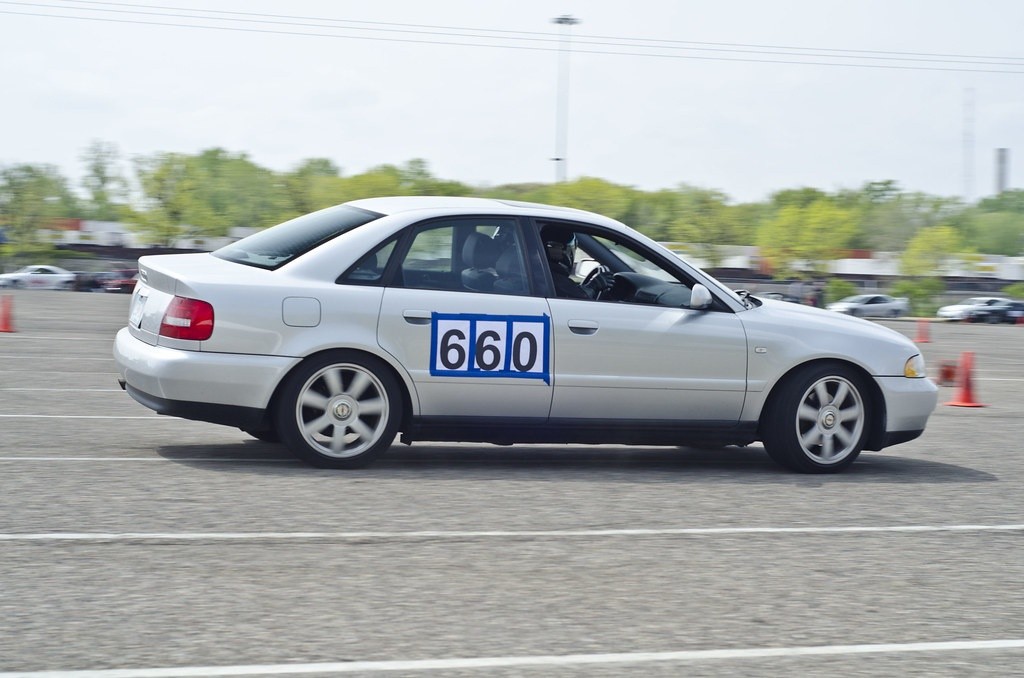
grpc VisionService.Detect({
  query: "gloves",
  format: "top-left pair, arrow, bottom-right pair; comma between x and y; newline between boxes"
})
579,271 -> 615,301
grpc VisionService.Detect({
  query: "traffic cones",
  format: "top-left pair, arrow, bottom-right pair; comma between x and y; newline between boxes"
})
0,293 -> 18,332
945,352 -> 991,408
911,319 -> 934,343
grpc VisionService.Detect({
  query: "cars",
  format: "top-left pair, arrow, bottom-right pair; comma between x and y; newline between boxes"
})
826,295 -> 909,318
0,265 -> 77,291
102,269 -> 139,293
964,300 -> 1024,323
936,297 -> 1014,322
753,292 -> 816,307
71,271 -> 111,292
111,196 -> 939,472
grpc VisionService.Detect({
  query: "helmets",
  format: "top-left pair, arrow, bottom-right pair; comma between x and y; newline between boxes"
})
540,225 -> 578,273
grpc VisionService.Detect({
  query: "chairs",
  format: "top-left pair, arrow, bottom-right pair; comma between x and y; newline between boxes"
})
460,231 -> 502,294
493,245 -> 526,295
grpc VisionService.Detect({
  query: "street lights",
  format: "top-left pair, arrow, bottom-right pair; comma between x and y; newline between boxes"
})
549,14 -> 582,184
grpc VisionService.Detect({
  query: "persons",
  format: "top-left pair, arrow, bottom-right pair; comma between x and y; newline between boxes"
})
540,224 -> 615,299
